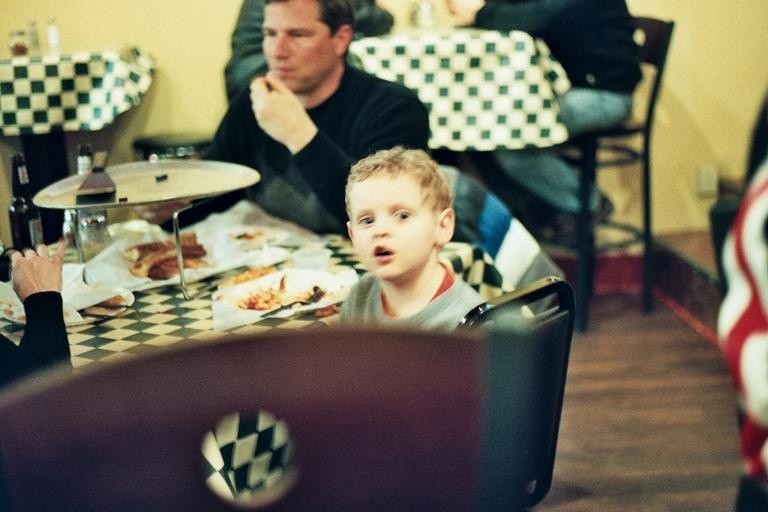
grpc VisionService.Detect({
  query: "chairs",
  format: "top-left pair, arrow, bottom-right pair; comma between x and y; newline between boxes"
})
518,14 -> 677,334
451,274 -> 577,509
0,305 -> 572,512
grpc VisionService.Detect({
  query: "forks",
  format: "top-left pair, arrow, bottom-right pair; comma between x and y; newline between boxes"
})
256,290 -> 327,319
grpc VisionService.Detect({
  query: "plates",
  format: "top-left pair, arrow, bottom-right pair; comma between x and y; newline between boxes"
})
0,265 -> 136,329
211,268 -> 359,333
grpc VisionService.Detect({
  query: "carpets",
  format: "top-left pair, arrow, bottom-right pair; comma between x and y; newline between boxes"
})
647,230 -> 722,345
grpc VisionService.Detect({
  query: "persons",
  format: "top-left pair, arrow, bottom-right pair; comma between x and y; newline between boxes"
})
717,88 -> 767,512
327,145 -> 491,338
452,0 -> 666,236
145,1 -> 425,231
0,239 -> 74,388
210,0 -> 394,104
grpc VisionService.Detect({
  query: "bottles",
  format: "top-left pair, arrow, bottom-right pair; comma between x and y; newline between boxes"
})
26,21 -> 39,60
46,19 -> 62,58
9,153 -> 44,253
76,143 -> 92,176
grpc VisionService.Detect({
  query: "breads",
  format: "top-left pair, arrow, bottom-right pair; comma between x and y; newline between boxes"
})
124,231 -> 208,280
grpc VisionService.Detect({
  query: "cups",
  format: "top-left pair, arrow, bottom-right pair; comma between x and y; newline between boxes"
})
8,32 -> 28,63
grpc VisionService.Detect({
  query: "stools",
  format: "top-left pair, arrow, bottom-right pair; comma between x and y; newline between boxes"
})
129,131 -> 208,158
